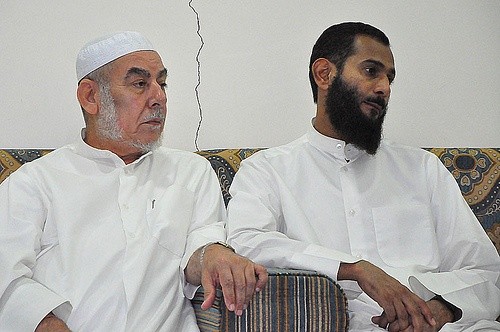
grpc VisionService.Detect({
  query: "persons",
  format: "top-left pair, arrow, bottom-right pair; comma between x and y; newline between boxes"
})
224,22 -> 499,331
0,30 -> 268,332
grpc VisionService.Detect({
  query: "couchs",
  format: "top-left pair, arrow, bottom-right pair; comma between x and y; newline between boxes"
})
1,148 -> 500,331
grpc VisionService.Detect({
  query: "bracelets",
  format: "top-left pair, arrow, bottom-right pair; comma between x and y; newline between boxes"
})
199,241 -> 236,266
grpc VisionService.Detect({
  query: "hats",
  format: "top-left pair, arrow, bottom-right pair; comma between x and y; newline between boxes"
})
76,30 -> 153,84
